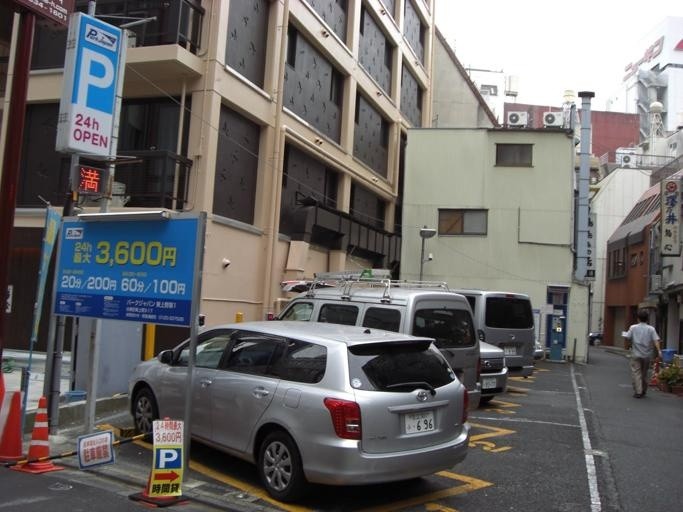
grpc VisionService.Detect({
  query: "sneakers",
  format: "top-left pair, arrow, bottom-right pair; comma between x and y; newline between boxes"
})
634,392 -> 647,399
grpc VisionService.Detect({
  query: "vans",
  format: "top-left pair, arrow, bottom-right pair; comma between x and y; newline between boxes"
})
277,269 -> 551,410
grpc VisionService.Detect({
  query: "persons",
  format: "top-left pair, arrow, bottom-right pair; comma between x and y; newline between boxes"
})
623,310 -> 665,398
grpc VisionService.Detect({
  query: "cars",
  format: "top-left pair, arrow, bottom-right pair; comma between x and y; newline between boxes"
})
589,330 -> 604,345
127,319 -> 472,501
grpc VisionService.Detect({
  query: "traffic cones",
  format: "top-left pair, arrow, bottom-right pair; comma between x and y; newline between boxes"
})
650,357 -> 659,386
1,390 -> 26,464
11,395 -> 65,475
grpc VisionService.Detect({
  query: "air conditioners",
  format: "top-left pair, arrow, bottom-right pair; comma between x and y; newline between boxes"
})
542,110 -> 564,128
506,109 -> 528,127
620,154 -> 637,169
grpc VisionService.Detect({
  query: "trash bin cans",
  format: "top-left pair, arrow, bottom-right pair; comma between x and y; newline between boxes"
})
661,349 -> 678,363
65,391 -> 86,402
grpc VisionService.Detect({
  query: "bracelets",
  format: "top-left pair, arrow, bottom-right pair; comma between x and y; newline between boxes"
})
658,350 -> 661,354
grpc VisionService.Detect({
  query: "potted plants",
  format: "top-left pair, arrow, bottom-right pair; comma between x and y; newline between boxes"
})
657,359 -> 683,394
